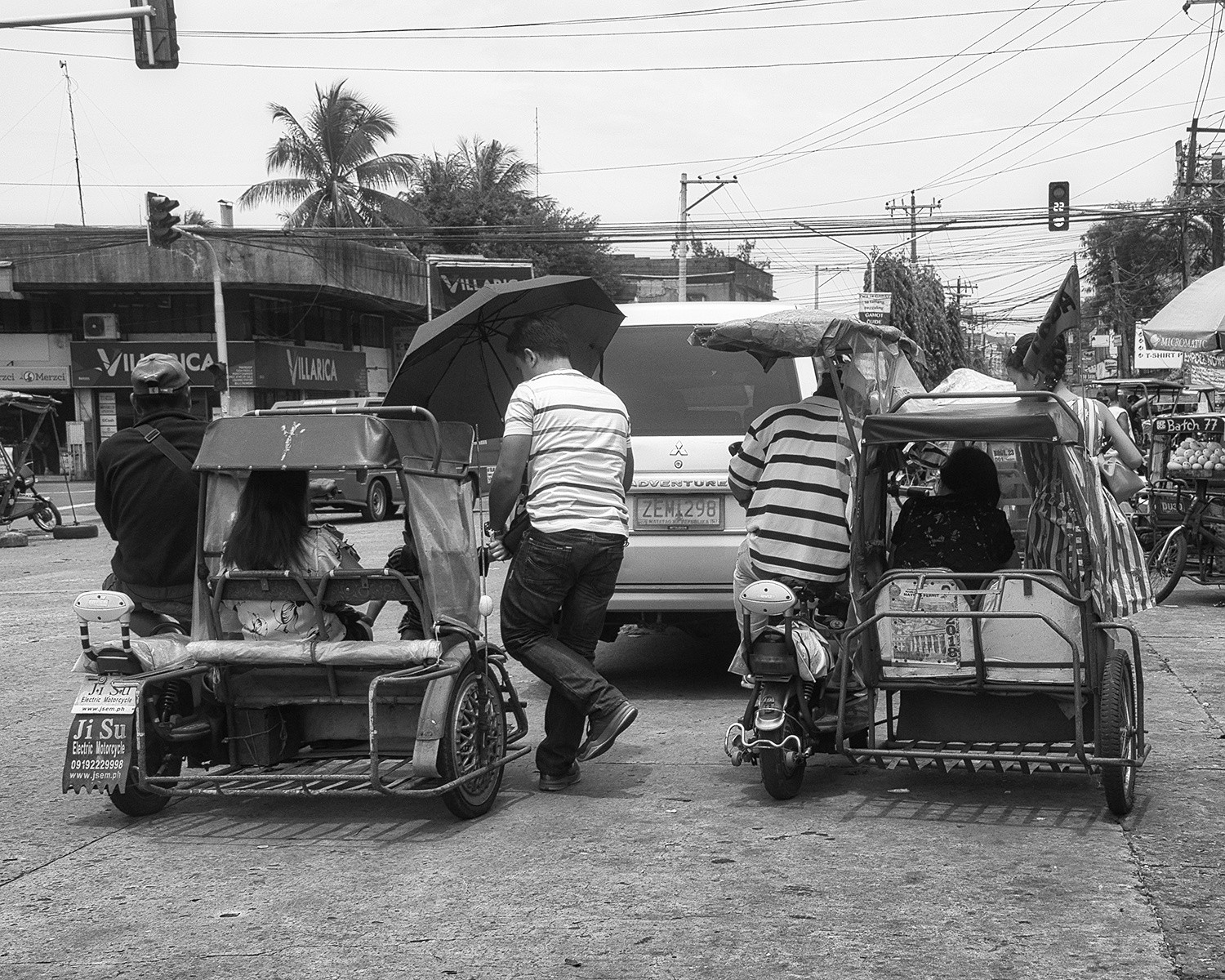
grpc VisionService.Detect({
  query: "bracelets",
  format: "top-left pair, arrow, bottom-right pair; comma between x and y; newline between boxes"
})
490,528 -> 504,538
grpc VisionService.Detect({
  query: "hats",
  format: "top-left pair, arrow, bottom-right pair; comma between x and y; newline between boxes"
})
131,353 -> 194,395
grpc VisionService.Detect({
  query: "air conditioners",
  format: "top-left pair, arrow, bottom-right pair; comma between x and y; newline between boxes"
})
82,311 -> 122,340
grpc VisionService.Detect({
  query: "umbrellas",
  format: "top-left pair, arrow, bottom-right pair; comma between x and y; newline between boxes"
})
1145,265 -> 1225,352
377,273 -> 626,440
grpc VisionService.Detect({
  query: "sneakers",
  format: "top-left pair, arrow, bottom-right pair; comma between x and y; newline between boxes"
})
539,761 -> 580,791
577,700 -> 638,762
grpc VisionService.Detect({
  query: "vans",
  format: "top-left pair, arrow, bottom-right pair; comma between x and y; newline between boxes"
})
587,299 -> 823,643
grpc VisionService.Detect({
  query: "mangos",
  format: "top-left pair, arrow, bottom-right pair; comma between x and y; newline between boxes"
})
1166,440 -> 1225,471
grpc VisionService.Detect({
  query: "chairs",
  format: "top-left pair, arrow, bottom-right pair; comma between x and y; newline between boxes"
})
744,380 -> 801,433
611,385 -> 690,436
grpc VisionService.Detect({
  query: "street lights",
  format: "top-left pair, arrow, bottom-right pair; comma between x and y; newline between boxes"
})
793,219 -> 959,292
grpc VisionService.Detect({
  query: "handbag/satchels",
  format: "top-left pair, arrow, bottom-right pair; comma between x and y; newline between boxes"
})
1088,400 -> 1145,504
501,510 -> 532,556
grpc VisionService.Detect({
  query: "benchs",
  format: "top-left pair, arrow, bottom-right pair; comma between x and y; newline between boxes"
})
856,566 -> 1092,702
184,566 -> 443,699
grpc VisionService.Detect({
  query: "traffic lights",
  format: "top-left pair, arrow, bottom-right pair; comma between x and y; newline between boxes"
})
146,190 -> 183,251
1049,181 -> 1069,232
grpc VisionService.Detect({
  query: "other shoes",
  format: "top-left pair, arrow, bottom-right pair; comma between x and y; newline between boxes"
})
741,674 -> 764,689
828,671 -> 866,690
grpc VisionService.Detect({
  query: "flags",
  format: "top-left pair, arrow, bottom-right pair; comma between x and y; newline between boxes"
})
1022,266 -> 1084,378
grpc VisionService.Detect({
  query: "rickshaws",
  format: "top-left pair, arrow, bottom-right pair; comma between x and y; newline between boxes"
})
1081,376 -> 1225,610
0,390 -> 62,533
720,388 -> 1165,819
62,397 -> 534,822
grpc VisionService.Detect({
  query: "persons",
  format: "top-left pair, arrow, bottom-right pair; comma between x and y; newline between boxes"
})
221,467 -> 508,644
726,368 -> 878,695
879,328 -> 1157,621
490,316 -> 640,790
94,353 -> 210,642
